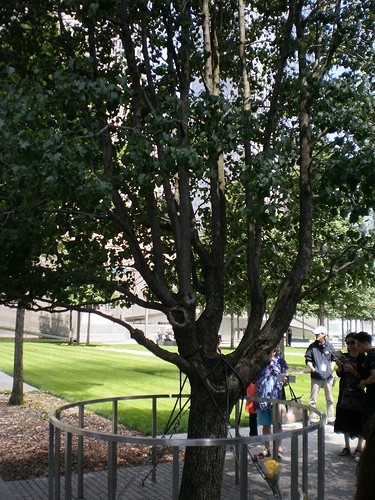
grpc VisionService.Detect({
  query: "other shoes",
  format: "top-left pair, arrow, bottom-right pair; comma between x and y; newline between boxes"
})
351,449 -> 362,458
277,450 -> 283,462
258,450 -> 271,458
339,447 -> 351,456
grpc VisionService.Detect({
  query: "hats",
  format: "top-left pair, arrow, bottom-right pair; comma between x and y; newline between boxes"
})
312,327 -> 327,334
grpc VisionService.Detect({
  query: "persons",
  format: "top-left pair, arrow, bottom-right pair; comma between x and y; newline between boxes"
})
334,331 -> 375,500
245,349 -> 289,461
304,326 -> 337,426
286,327 -> 292,346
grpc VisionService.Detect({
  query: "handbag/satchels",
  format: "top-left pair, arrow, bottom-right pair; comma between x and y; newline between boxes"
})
272,382 -> 304,424
320,377 -> 335,388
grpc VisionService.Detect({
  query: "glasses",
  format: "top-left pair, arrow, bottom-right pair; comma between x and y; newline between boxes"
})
346,340 -> 357,345
